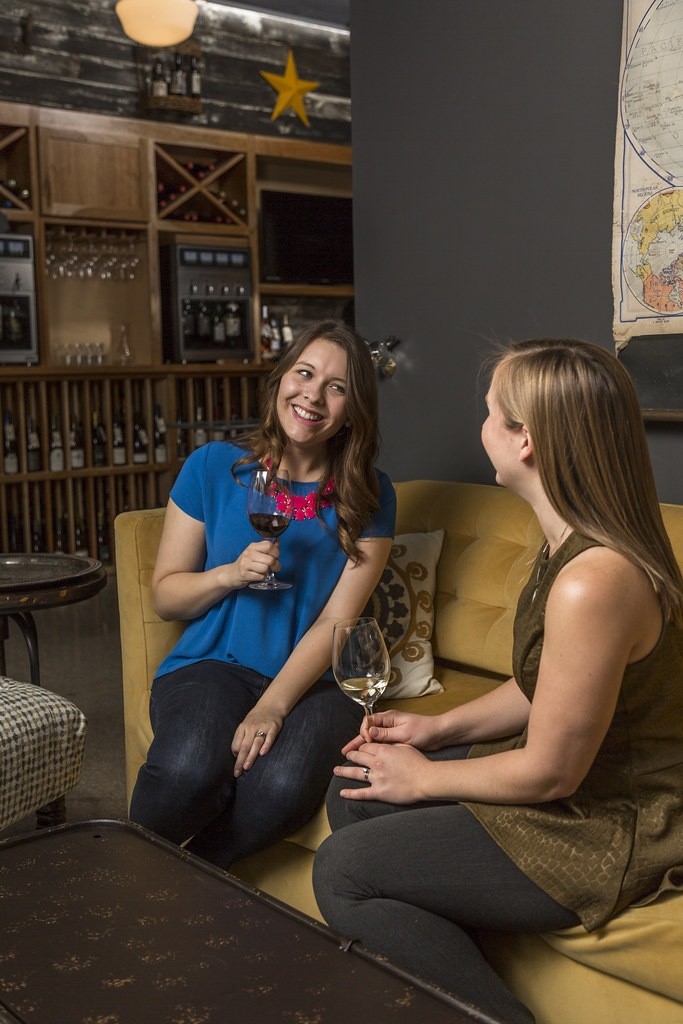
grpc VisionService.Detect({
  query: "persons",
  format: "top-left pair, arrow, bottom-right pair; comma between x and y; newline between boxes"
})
127,318 -> 398,870
312,337 -> 683,1024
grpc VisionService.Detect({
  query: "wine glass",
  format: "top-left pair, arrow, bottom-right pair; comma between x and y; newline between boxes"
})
332,616 -> 390,743
44,225 -> 141,281
246,468 -> 295,590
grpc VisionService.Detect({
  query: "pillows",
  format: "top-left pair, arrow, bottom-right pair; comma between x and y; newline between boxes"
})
363,529 -> 444,698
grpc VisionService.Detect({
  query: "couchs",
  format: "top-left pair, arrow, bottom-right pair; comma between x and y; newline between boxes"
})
113,482 -> 683,1024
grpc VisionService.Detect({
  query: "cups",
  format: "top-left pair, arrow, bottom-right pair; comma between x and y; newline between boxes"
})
50,342 -> 104,366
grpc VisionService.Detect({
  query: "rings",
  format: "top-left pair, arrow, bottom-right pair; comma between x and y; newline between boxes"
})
363,768 -> 369,779
255,731 -> 266,739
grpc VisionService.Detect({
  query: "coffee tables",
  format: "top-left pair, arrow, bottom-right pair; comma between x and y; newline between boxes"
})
0,818 -> 506,1024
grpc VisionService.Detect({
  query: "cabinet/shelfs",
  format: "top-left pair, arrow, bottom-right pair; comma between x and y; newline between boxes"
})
0,119 -> 354,571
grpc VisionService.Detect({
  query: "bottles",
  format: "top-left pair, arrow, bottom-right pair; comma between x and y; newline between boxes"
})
187,57 -> 202,98
154,159 -> 247,222
0,178 -> 31,209
169,52 -> 187,95
0,507 -> 129,567
0,408 -> 260,475
0,306 -> 31,350
181,297 -> 247,351
113,323 -> 135,364
149,56 -> 168,97
258,303 -> 295,367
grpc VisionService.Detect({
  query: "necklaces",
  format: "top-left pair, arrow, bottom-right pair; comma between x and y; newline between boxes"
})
530,524 -> 568,602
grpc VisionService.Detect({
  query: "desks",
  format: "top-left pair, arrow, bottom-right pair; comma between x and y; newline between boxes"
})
0,568 -> 108,687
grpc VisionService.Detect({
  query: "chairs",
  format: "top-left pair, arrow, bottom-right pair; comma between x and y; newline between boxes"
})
0,675 -> 89,836
